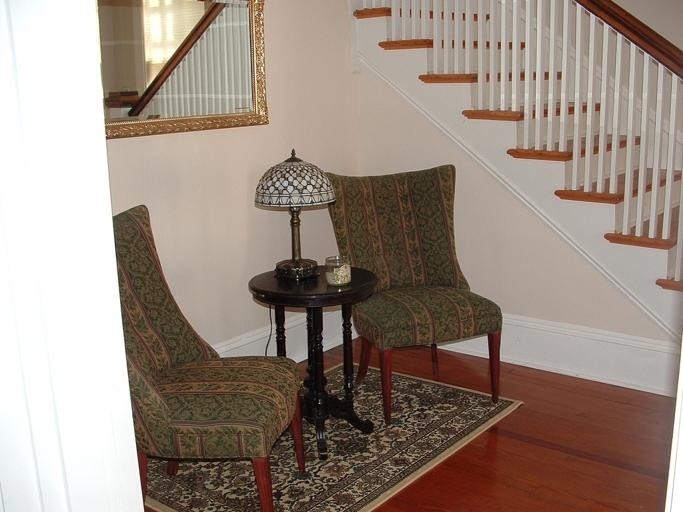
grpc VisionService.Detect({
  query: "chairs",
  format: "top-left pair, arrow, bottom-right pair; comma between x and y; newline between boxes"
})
113,203 -> 307,512
319,162 -> 505,425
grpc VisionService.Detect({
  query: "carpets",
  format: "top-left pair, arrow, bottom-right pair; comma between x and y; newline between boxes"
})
146,360 -> 527,512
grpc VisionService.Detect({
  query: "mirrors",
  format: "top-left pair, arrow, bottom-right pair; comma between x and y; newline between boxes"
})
98,0 -> 270,142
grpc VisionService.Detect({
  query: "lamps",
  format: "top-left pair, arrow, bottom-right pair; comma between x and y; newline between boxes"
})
252,147 -> 338,283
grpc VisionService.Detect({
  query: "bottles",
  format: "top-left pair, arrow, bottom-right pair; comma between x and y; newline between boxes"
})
323,256 -> 353,286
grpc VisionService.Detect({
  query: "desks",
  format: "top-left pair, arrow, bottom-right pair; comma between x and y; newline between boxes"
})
249,264 -> 380,462
